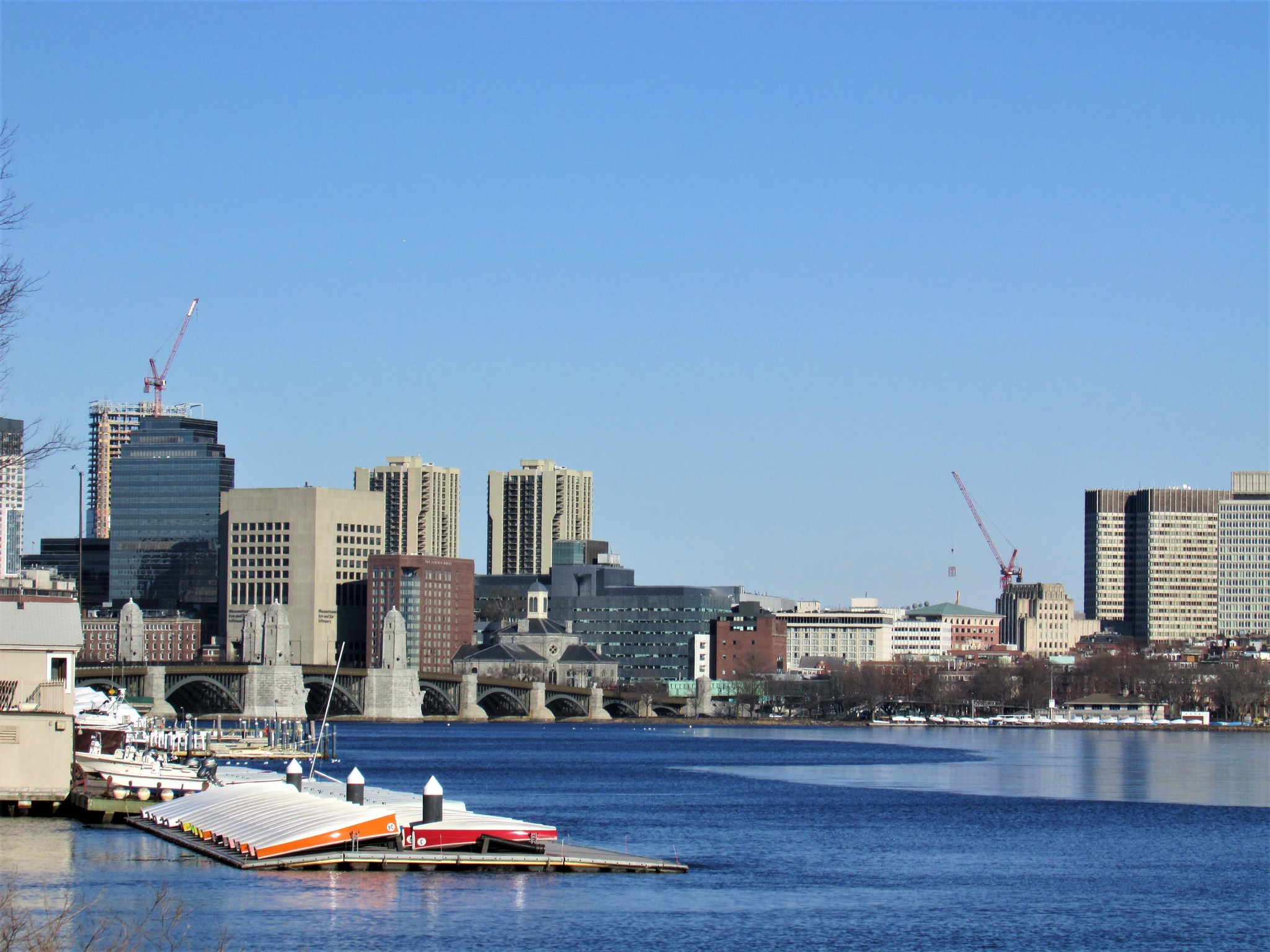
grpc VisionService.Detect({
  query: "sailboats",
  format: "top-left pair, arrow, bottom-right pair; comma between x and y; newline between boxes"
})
74,642 -> 347,790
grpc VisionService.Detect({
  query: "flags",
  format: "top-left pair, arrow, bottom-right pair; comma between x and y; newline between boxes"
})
32,542 -> 35,544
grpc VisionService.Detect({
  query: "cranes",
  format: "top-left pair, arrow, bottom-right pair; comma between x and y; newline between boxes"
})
951,471 -> 1023,589
143,297 -> 199,416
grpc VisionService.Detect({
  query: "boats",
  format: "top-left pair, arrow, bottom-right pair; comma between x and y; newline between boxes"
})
944,717 -> 959,722
990,716 -> 1018,722
929,717 -> 943,722
892,716 -> 908,721
873,720 -> 890,723
1119,719 -> 1135,723
1170,719 -> 1186,724
959,717 -> 989,723
1103,719 -> 1117,723
1052,718 -> 1100,723
1019,717 -> 1051,723
910,717 -> 926,721
1187,719 -> 1202,724
1137,719 -> 1153,724
1155,719 -> 1170,724
74,661 -> 144,753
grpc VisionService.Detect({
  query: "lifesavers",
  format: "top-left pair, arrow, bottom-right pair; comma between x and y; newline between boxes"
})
264,728 -> 272,735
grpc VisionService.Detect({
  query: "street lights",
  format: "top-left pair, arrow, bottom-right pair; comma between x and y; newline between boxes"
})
180,707 -> 184,729
